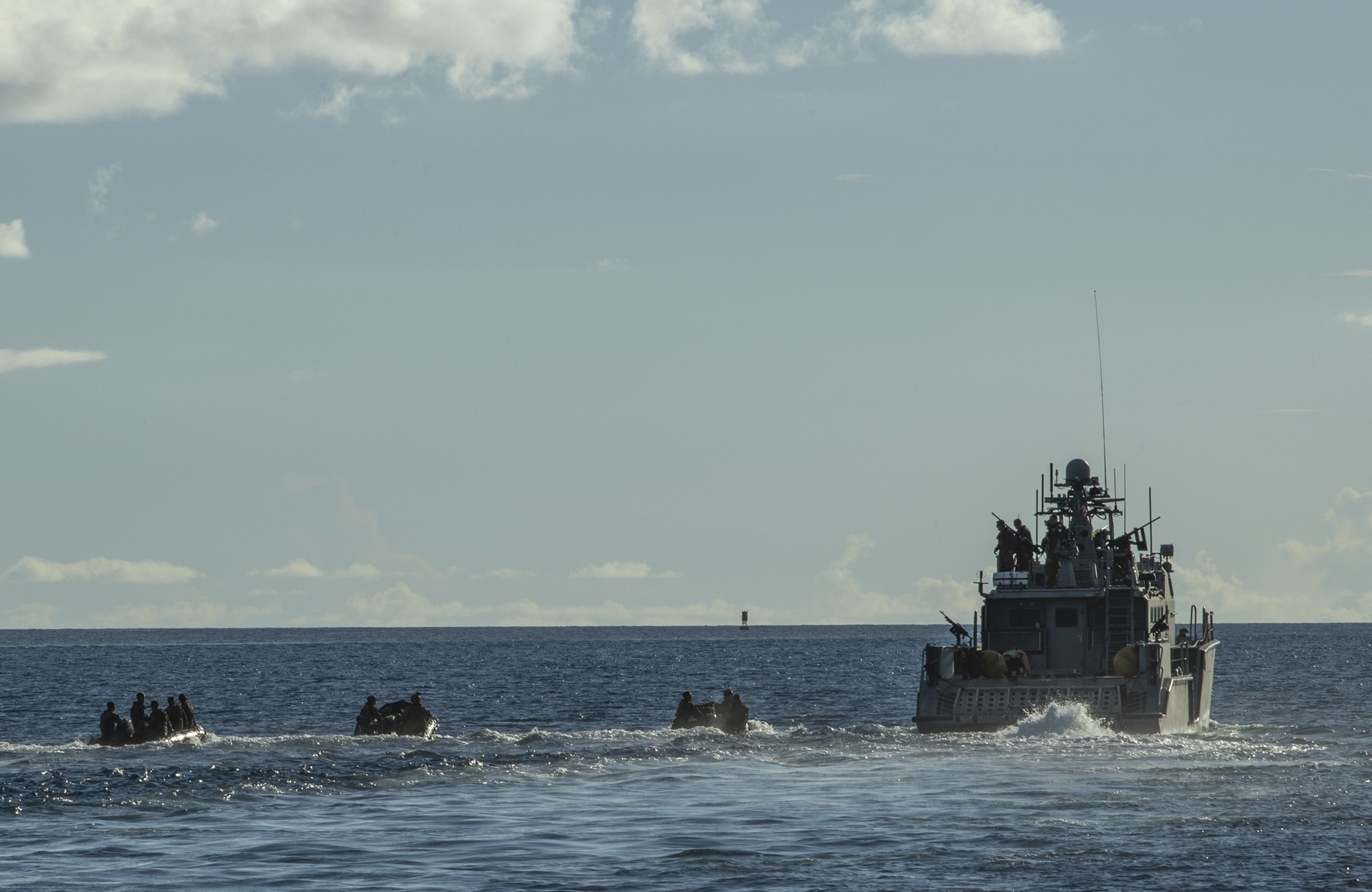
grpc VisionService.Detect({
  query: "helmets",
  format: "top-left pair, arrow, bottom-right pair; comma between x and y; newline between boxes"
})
411,694 -> 420,702
1045,520 -> 1057,527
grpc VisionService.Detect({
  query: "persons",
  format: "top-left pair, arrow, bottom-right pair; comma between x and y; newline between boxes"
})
99,692 -> 195,741
359,694 -> 430,736
994,518 -> 1034,572
1041,515 -> 1067,588
675,688 -> 747,730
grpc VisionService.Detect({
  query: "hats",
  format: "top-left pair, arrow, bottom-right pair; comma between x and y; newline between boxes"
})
178,693 -> 188,700
723,689 -> 734,695
682,691 -> 690,698
367,696 -> 375,701
733,694 -> 740,698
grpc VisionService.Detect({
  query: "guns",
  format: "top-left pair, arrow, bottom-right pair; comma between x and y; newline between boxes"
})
1147,610 -> 1169,642
1103,516 -> 1161,549
939,610 -> 973,646
991,511 -> 1045,554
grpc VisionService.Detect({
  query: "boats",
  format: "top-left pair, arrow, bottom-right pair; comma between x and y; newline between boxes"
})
87,723 -> 205,747
911,290 -> 1221,735
354,700 -> 439,739
672,702 -> 750,735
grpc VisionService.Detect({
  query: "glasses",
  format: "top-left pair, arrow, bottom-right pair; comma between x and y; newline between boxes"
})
1015,523 -> 1018,526
150,706 -> 153,707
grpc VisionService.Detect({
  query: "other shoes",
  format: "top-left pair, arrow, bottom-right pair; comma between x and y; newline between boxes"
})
1044,584 -> 1054,589
